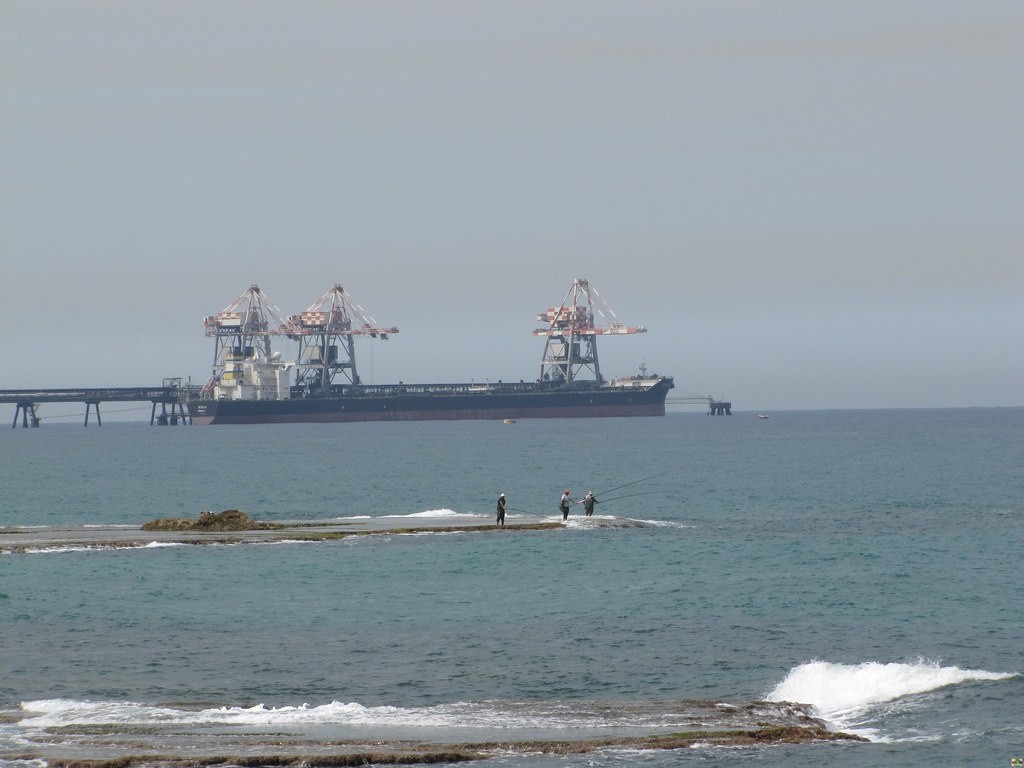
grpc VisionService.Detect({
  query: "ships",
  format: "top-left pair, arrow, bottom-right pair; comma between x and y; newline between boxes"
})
186,273 -> 677,427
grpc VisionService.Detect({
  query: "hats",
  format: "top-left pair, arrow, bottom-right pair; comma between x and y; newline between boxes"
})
565,490 -> 570,495
588,490 -> 593,494
500,494 -> 505,497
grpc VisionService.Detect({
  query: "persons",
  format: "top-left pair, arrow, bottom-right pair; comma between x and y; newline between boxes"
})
585,490 -> 600,516
496,493 -> 506,525
560,490 -> 576,519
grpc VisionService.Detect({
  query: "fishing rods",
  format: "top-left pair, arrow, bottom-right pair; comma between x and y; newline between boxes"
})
504,506 -> 552,523
599,489 -> 674,503
571,470 -> 666,504
